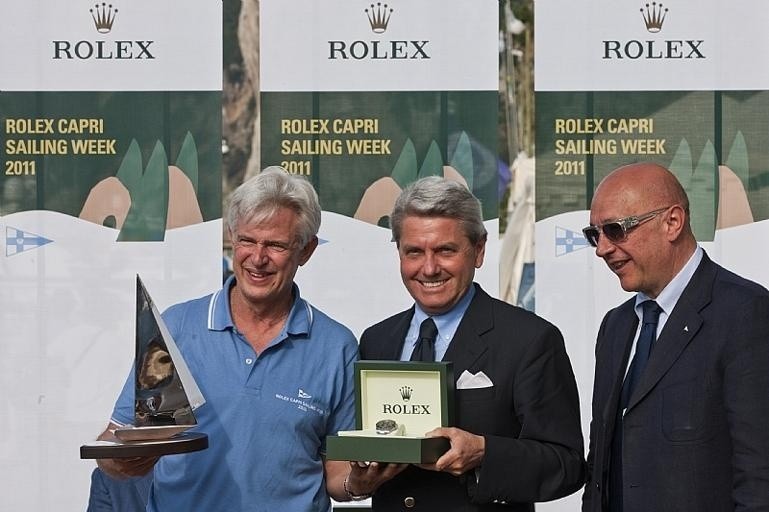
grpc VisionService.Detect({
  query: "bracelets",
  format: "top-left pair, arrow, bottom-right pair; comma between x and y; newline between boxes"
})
343,475 -> 374,502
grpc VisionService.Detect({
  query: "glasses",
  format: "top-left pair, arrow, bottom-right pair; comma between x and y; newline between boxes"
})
582,208 -> 667,247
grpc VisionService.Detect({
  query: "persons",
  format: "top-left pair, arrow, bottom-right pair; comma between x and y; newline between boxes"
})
86,165 -> 409,512
356,174 -> 585,510
583,162 -> 768,512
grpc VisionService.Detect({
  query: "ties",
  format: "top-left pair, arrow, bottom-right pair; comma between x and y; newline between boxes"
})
621,302 -> 664,405
410,320 -> 438,361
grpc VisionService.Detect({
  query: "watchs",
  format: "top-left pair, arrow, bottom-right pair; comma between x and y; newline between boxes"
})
375,420 -> 398,435
